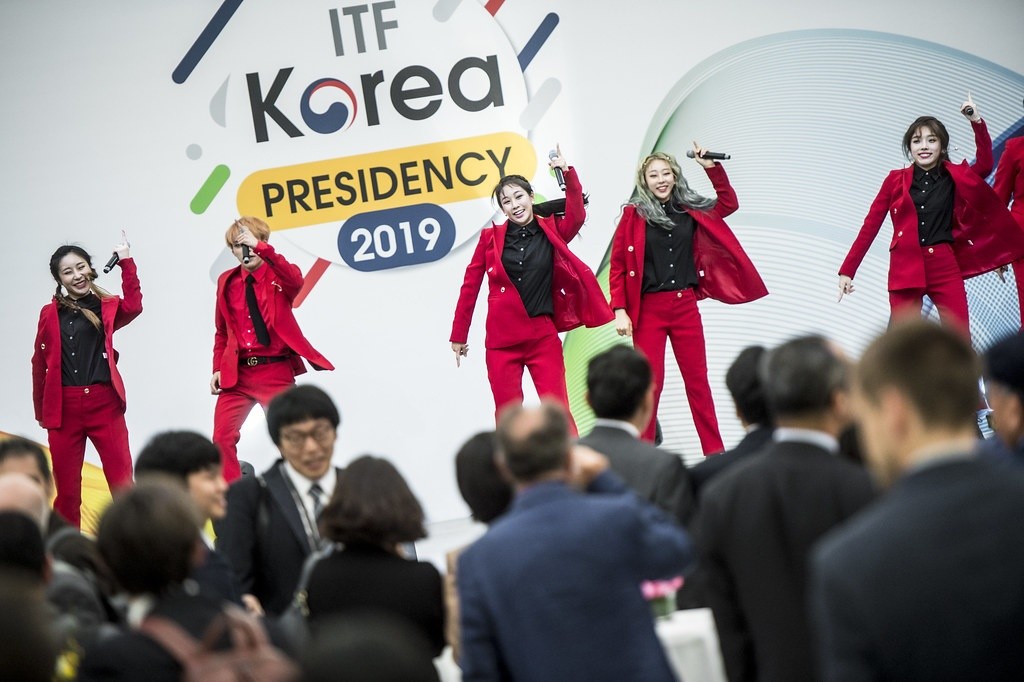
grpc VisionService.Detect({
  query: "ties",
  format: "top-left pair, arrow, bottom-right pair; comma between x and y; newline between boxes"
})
245,274 -> 271,347
308,484 -> 325,541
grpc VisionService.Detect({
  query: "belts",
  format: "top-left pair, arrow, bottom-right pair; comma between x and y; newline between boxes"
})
238,355 -> 290,366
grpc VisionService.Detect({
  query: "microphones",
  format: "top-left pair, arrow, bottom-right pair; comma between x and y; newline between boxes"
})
964,106 -> 973,115
687,150 -> 731,159
103,242 -> 130,274
240,226 -> 250,263
549,150 -> 567,191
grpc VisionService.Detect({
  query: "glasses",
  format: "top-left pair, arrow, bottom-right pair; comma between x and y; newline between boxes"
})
282,423 -> 334,447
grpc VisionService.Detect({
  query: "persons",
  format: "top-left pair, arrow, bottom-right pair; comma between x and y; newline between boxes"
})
33,228 -> 143,534
450,142 -> 615,440
211,216 -> 336,484
0,320 -> 1024,682
610,138 -> 769,459
837,90 -> 994,348
991,97 -> 1024,335
